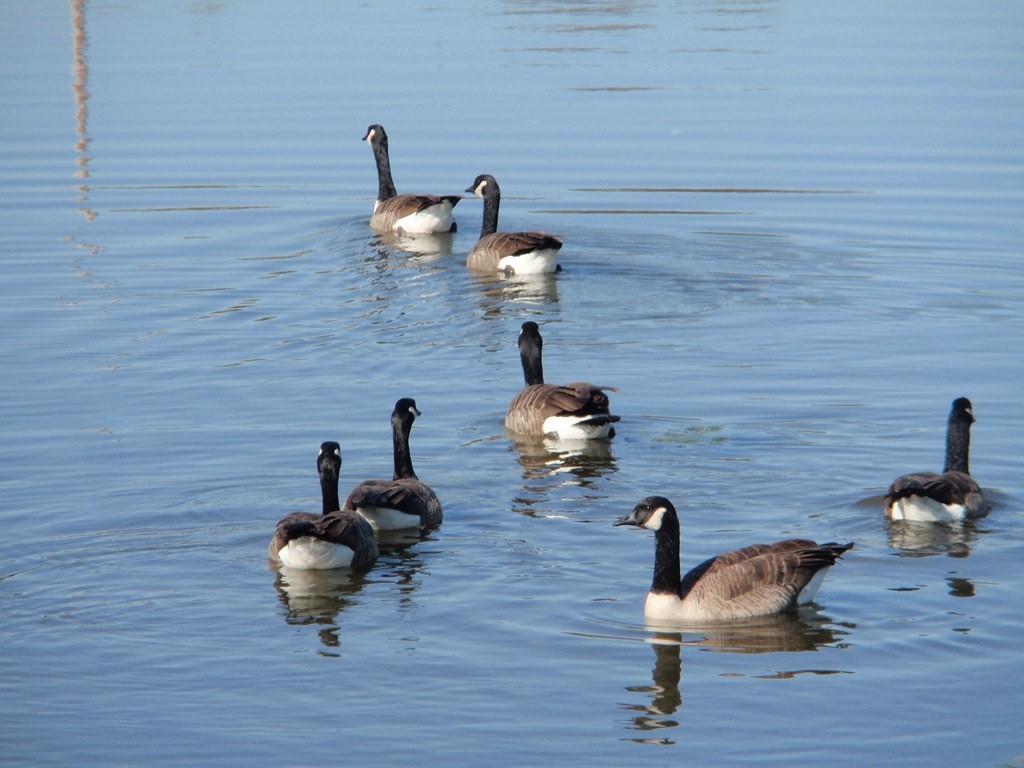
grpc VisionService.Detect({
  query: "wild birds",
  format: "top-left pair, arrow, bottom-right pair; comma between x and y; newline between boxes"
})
504,321 -> 620,440
342,398 -> 441,534
362,125 -> 467,240
267,443 -> 378,571
884,398 -> 986,522
468,173 -> 565,277
614,496 -> 855,630
